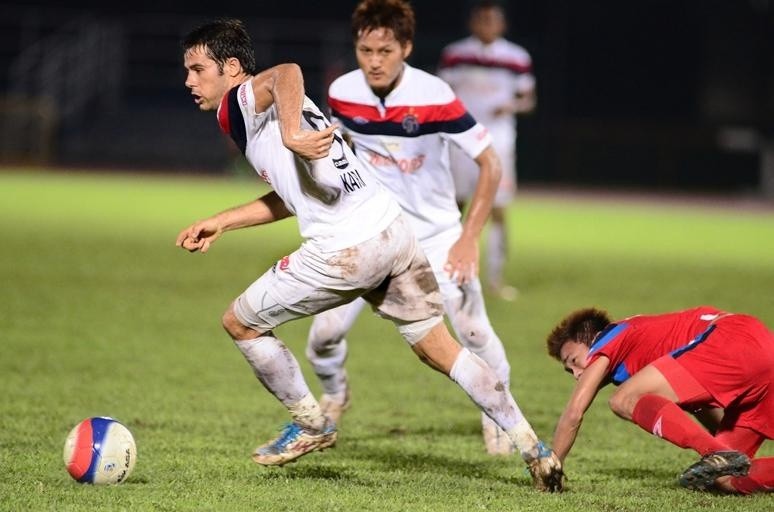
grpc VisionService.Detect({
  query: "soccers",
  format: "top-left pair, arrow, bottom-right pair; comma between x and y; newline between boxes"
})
62,417 -> 138,485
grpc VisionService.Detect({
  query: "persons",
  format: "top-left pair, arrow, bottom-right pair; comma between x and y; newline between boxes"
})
304,0 -> 518,458
436,2 -> 541,303
547,304 -> 773,495
174,16 -> 568,494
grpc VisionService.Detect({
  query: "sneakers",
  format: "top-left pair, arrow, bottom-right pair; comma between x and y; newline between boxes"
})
251,415 -> 338,466
679,450 -> 752,491
520,441 -> 567,493
480,409 -> 517,457
318,388 -> 352,427
